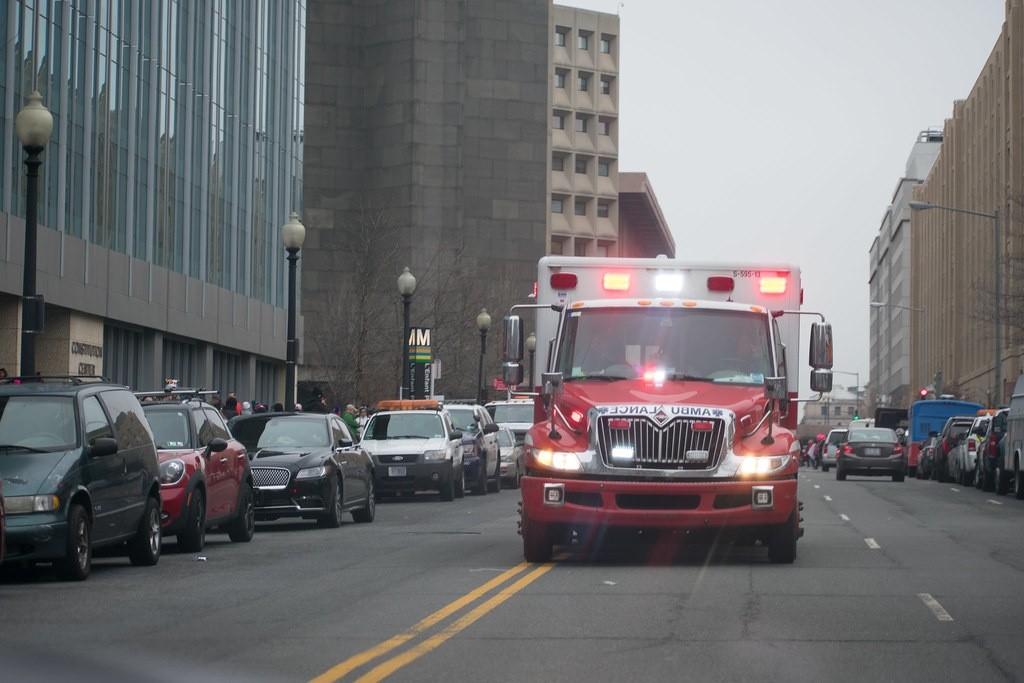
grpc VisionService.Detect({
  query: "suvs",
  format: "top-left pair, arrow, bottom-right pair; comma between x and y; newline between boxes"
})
0,374 -> 165,585
130,387 -> 258,550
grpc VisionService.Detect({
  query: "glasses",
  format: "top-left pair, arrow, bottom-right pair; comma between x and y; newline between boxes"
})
360,411 -> 366,413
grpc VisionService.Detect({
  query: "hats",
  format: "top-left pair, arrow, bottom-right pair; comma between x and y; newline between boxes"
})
359,406 -> 367,412
242,402 -> 250,409
346,403 -> 357,411
313,386 -> 323,399
226,398 -> 238,411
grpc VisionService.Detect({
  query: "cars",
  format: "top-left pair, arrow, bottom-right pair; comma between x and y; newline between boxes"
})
225,412 -> 376,530
848,372 -> 1024,499
358,402 -> 465,501
818,428 -> 847,472
439,397 -> 526,495
834,427 -> 906,482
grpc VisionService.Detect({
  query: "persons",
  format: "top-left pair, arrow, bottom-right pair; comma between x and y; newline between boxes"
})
295,404 -> 303,413
142,396 -> 155,401
332,404 -> 379,444
803,439 -> 816,467
304,386 -> 331,413
0,367 -> 7,378
209,392 -> 269,420
269,403 -> 283,412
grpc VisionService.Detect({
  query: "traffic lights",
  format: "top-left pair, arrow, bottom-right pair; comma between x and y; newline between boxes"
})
921,388 -> 927,400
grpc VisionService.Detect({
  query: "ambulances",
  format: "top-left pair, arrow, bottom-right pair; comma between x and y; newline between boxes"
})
501,257 -> 834,565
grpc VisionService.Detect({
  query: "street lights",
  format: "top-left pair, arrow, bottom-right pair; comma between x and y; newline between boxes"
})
831,369 -> 860,419
15,91 -> 54,379
525,331 -> 536,393
477,307 -> 491,406
396,266 -> 417,400
871,302 -> 926,391
283,211 -> 305,411
906,200 -> 1000,408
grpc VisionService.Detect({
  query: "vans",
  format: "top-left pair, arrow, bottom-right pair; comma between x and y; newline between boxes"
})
481,398 -> 535,443
905,399 -> 984,478
